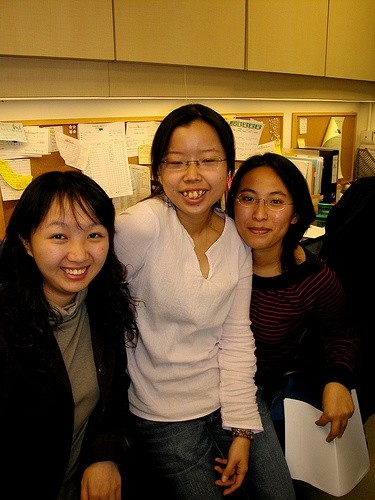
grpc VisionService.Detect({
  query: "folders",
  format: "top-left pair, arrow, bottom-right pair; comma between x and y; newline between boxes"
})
319,149 -> 340,203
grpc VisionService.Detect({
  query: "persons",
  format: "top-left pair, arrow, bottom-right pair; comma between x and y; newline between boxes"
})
0,170 -> 138,500
225,153 -> 356,500
114,103 -> 295,500
325,176 -> 375,429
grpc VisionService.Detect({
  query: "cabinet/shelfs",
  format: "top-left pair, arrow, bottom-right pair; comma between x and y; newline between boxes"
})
0,0 -> 375,82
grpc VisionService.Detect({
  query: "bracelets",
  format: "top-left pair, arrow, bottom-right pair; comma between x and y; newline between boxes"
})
232,427 -> 253,440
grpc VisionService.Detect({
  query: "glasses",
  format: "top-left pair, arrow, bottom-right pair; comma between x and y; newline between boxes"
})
233,191 -> 294,212
162,155 -> 228,171
